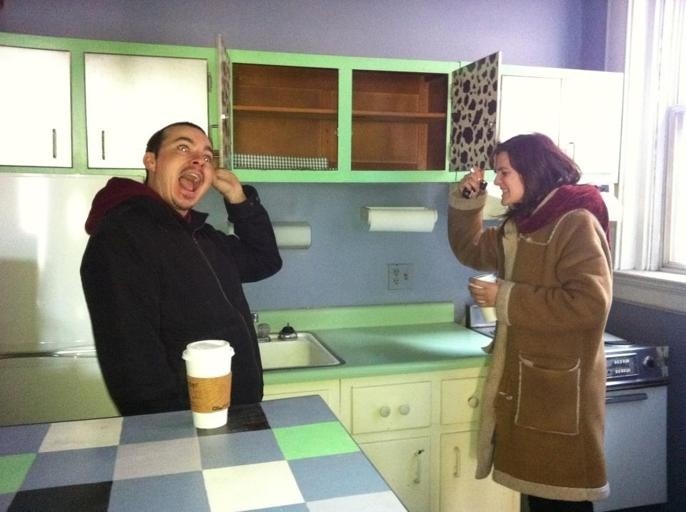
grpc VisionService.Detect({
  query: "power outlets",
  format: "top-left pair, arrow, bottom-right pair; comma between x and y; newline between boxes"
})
388,263 -> 416,289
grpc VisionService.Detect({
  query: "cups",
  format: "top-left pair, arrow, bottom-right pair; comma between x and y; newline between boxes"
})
182,339 -> 236,430
472,273 -> 498,323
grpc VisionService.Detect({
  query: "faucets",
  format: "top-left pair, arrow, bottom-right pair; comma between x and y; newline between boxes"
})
250,308 -> 269,342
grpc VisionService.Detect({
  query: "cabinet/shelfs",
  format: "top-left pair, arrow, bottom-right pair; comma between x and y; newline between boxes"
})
340,367 -> 522,512
261,379 -> 340,421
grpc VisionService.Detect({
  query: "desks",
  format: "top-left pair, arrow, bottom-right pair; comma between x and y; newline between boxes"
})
0,395 -> 409,512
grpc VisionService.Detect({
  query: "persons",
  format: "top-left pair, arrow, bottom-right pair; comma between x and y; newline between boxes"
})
80,123 -> 282,414
447,133 -> 616,512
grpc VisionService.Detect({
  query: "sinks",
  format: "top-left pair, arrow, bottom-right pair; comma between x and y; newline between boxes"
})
256,330 -> 344,370
